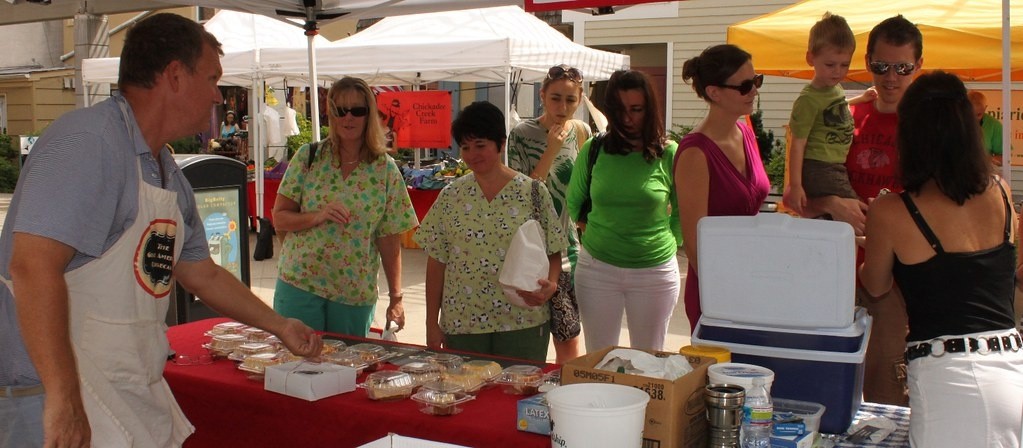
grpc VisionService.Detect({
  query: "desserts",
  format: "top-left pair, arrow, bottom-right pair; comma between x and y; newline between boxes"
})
211,324 -> 540,415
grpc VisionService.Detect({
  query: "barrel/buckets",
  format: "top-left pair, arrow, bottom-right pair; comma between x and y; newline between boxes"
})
545,382 -> 650,448
706,363 -> 775,421
680,345 -> 731,364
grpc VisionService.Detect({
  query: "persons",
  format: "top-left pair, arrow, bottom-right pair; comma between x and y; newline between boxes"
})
508,64 -> 593,363
783,14 -> 925,405
568,68 -> 683,354
859,69 -> 1023,448
788,14 -> 878,223
273,76 -> 419,337
673,44 -> 770,335
410,100 -> 570,363
219,110 -> 240,138
1,13 -> 324,448
967,91 -> 1003,167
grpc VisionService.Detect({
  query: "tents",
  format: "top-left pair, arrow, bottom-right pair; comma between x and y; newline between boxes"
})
726,1 -> 1023,81
261,6 -> 630,227
80,7 -> 330,236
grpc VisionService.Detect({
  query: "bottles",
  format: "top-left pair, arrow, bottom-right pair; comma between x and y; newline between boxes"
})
741,376 -> 774,448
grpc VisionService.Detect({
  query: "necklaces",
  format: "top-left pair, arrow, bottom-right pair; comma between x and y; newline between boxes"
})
340,160 -> 358,165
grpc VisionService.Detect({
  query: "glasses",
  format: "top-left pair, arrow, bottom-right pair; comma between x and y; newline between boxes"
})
867,55 -> 919,75
331,106 -> 369,117
710,74 -> 764,95
545,66 -> 584,83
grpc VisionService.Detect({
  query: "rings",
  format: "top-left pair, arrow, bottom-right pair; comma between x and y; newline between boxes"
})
559,133 -> 563,137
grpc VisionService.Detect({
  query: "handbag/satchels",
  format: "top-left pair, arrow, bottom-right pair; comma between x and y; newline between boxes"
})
578,130 -> 605,223
532,179 -> 582,343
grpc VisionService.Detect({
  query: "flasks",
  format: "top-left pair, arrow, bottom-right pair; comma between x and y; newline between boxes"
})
703,383 -> 746,448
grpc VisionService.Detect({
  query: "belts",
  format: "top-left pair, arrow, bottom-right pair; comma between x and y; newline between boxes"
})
0,385 -> 46,398
904,333 -> 1022,365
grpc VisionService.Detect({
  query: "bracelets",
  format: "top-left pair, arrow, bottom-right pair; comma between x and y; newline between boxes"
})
532,170 -> 546,184
388,291 -> 403,298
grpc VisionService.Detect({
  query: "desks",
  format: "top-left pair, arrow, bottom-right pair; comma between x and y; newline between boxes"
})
398,185 -> 444,250
162,315 -> 563,448
820,402 -> 915,448
246,177 -> 284,236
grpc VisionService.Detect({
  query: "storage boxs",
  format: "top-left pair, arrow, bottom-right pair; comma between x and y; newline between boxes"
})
517,392 -> 553,436
560,345 -> 718,448
689,212 -> 874,434
769,397 -> 826,448
264,359 -> 356,401
